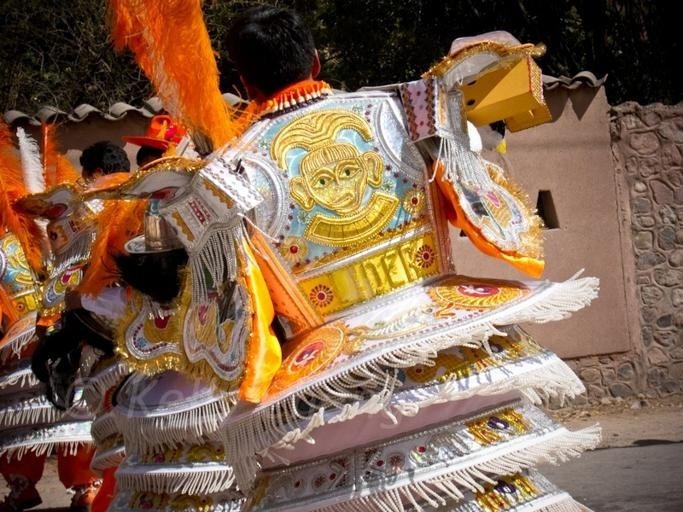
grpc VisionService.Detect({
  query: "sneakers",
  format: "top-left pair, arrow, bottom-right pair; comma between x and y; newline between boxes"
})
1,481 -> 43,512
71,488 -> 96,509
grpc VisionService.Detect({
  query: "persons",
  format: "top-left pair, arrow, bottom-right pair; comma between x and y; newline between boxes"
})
0,113 -> 239,511
115,0 -> 601,512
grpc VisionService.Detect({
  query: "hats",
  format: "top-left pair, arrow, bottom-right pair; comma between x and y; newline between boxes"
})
121,115 -> 172,150
